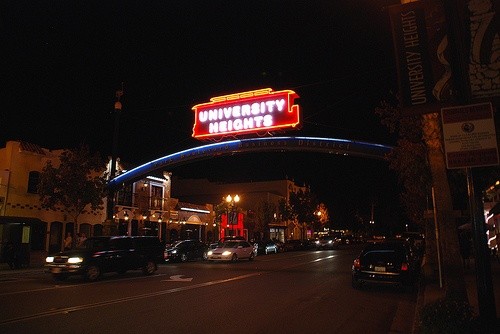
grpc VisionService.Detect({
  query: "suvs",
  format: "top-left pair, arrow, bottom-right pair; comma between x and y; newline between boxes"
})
43,236 -> 162,282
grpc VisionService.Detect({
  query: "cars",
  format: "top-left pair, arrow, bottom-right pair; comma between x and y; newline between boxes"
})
352,244 -> 420,292
313,235 -> 339,251
163,237 -> 279,263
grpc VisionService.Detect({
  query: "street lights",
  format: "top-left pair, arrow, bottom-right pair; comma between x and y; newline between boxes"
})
102,79 -> 124,239
225,194 -> 241,239
